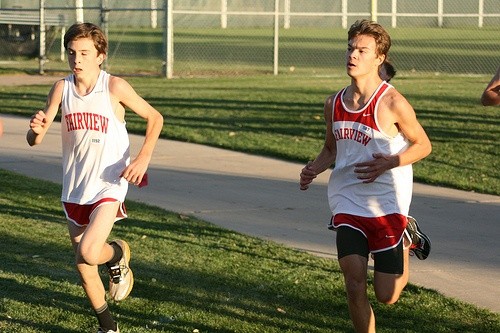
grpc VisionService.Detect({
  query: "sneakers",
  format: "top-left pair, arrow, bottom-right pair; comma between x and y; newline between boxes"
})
101,239 -> 134,302
99,320 -> 123,333
404,215 -> 431,260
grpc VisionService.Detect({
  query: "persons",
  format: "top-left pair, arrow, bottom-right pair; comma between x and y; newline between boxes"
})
300,19 -> 432,333
27,21 -> 164,333
